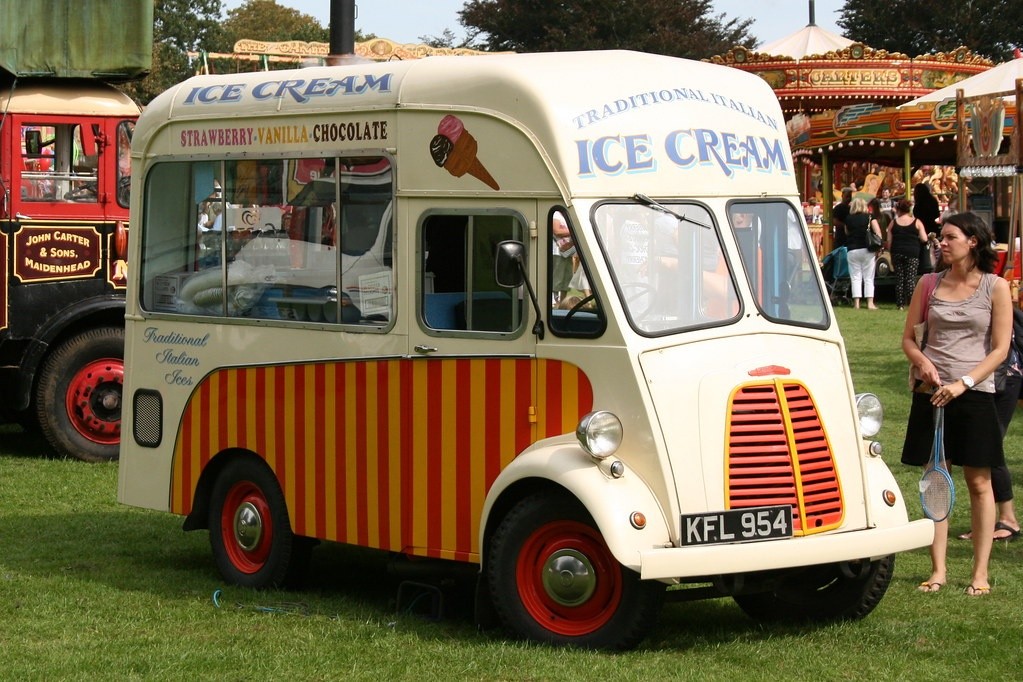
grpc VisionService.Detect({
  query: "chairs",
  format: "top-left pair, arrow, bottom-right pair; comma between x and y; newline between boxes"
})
422,292 -> 524,333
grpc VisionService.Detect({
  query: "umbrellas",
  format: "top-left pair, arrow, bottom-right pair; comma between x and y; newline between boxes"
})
896,50 -> 1023,111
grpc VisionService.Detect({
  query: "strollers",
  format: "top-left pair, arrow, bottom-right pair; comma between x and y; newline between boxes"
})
793,244 -> 855,307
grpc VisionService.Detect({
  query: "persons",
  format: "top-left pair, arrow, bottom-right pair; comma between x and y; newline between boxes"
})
805,181 -> 958,309
197,188 -> 257,258
969,177 -> 995,233
901,211 -> 1023,594
49,134 -> 81,188
26,160 -> 51,200
551,210 -> 800,322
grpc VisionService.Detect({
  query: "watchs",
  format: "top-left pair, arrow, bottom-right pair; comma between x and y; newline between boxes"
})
962,376 -> 974,389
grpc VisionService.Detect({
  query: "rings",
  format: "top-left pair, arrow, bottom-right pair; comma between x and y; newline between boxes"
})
941,393 -> 945,397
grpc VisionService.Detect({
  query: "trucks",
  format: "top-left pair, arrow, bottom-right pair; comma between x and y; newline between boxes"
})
0,82 -> 147,465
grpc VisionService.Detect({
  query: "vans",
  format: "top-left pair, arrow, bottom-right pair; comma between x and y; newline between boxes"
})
115,49 -> 935,657
227,175 -> 392,324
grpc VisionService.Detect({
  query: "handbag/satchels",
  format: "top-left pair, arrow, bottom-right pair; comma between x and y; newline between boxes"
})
908,272 -> 938,391
866,215 -> 882,252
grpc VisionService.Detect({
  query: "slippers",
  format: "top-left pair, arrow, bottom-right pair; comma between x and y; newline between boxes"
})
993,522 -> 1021,540
968,584 -> 990,592
958,530 -> 973,539
921,582 -> 942,589
